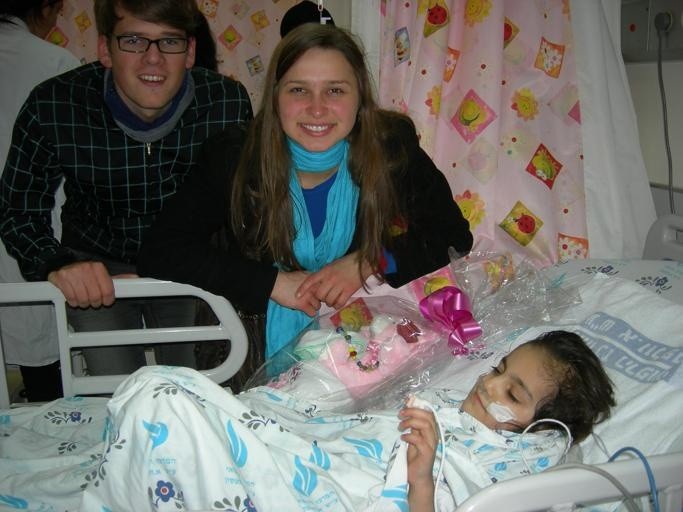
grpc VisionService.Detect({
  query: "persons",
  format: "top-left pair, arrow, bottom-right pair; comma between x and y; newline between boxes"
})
395,328 -> 617,512
1,1 -> 269,396
136,23 -> 473,396
1,0 -> 84,404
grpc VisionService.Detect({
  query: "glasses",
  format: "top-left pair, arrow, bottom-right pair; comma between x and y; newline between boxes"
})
106,31 -> 190,55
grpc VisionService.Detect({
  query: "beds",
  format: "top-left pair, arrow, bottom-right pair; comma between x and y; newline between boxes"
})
0,214 -> 683,512
